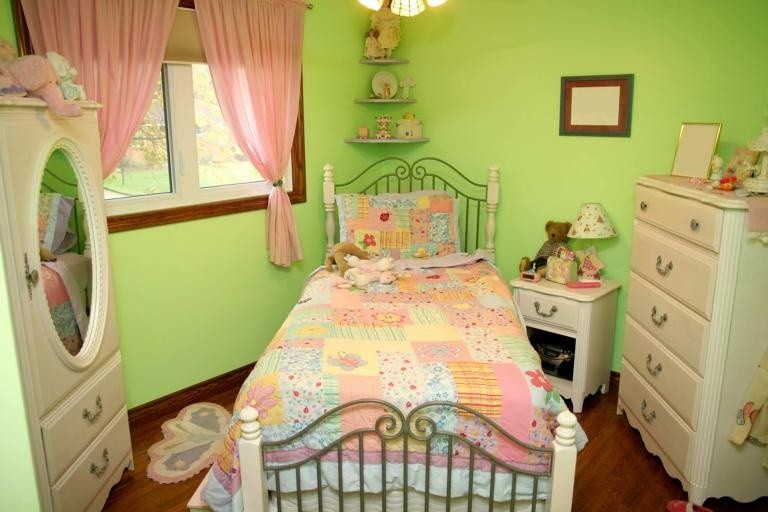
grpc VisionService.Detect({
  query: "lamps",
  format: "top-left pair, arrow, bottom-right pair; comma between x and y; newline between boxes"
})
358,0 -> 449,19
566,203 -> 619,284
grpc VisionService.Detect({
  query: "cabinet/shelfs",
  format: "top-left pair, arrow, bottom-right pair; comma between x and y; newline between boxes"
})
509,273 -> 622,413
616,176 -> 768,510
342,57 -> 431,145
0,92 -> 135,511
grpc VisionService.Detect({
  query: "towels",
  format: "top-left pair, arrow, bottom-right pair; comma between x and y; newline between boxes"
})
727,349 -> 768,470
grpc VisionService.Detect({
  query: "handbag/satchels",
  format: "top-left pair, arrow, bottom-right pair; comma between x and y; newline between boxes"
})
545,245 -> 579,285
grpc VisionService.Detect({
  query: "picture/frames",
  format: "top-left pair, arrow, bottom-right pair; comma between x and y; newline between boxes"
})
670,121 -> 722,180
753,150 -> 768,181
723,148 -> 760,178
559,74 -> 634,139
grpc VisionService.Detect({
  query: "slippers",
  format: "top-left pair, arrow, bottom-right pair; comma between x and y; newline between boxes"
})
667,499 -> 713,512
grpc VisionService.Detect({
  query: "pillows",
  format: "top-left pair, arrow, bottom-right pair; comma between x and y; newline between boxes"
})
333,191 -> 462,256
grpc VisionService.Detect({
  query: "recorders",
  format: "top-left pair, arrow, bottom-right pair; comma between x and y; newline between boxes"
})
532,338 -> 574,382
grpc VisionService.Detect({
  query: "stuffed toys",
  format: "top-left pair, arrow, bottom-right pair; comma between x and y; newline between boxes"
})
710,156 -> 723,181
0,39 -> 84,117
519,220 -> 574,273
364,0 -> 402,59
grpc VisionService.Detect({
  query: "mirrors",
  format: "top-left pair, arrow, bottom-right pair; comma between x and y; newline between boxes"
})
31,135 -> 110,372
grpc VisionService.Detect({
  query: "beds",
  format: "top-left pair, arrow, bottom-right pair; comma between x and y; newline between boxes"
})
202,157 -> 589,512
